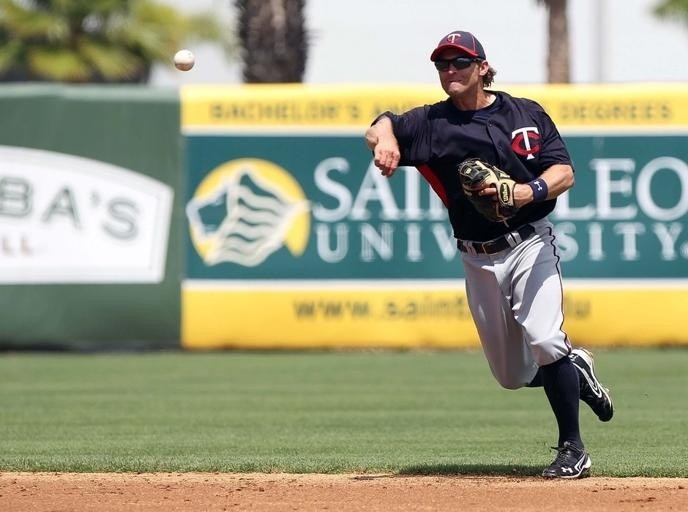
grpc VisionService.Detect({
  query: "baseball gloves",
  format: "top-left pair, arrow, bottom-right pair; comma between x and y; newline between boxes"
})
457,156 -> 519,222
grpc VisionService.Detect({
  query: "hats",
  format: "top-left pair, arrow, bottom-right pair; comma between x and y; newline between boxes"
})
429,30 -> 487,63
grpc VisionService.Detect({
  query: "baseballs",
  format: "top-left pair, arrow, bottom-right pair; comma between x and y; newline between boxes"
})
172,49 -> 196,71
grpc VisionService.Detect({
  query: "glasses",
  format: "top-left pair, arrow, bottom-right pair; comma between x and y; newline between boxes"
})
434,57 -> 481,72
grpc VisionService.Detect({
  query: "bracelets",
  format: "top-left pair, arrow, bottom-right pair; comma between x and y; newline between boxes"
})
526,176 -> 549,203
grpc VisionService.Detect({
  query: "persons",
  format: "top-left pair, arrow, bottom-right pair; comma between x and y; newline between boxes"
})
363,28 -> 615,480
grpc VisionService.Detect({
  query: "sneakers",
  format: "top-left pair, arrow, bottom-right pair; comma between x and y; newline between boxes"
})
542,440 -> 595,480
567,343 -> 617,422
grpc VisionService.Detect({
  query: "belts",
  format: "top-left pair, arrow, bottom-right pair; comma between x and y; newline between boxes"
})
452,221 -> 537,254
